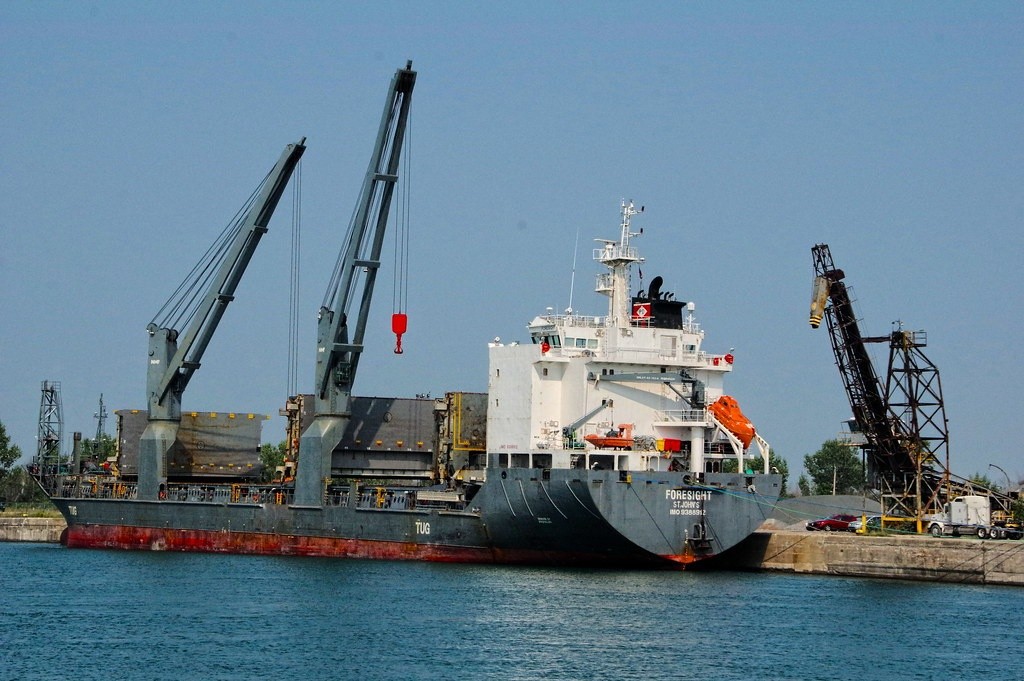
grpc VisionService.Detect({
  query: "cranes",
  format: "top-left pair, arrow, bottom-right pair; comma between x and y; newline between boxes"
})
809,242 -> 959,536
134,135 -> 310,500
290,57 -> 418,500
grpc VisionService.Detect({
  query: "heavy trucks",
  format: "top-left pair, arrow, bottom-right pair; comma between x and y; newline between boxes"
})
927,496 -> 1024,541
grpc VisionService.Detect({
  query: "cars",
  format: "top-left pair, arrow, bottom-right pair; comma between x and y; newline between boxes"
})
806,513 -> 857,532
848,514 -> 882,533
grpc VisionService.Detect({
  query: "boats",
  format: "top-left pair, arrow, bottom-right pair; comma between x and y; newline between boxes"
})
583,434 -> 634,448
25,44 -> 785,572
708,396 -> 756,449
